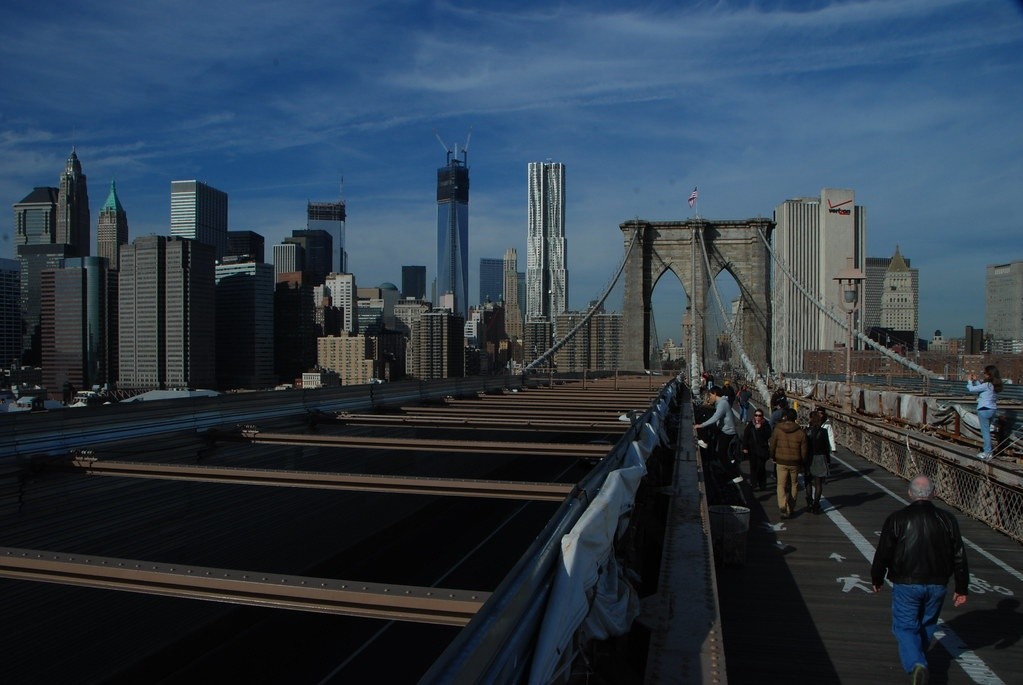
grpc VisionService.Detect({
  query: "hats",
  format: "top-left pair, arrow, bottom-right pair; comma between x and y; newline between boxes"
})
817,406 -> 826,411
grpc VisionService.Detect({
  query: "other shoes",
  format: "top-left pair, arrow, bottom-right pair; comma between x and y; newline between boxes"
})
977,452 -> 993,459
698,440 -> 708,448
913,665 -> 927,685
728,476 -> 744,485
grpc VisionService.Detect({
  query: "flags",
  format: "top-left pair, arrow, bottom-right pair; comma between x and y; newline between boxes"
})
688,187 -> 698,208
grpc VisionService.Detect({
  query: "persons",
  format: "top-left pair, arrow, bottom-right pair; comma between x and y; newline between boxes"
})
966,365 -> 1004,459
871,473 -> 969,685
691,371 -> 837,520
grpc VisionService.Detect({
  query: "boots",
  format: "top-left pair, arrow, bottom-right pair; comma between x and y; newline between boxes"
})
807,497 -> 821,515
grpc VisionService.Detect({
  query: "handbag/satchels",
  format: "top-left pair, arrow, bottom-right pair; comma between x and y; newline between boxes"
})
810,454 -> 829,478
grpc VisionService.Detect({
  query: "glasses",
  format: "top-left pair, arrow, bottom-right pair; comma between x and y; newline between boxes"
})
754,414 -> 763,418
